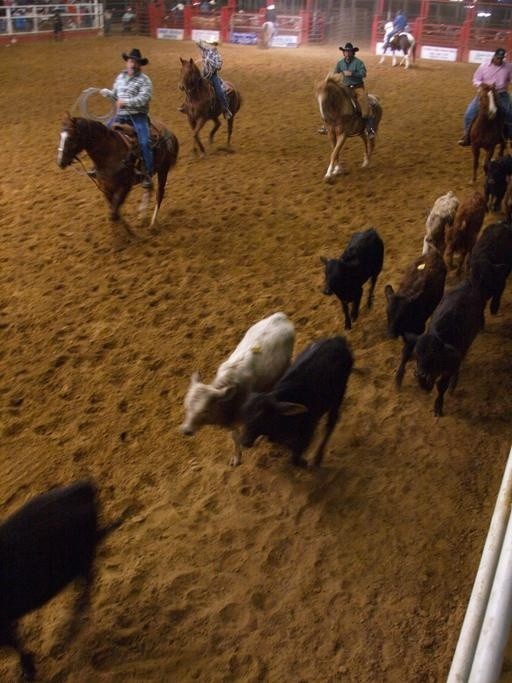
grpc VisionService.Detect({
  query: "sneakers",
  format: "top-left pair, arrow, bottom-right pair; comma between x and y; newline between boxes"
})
367,128 -> 376,139
318,124 -> 328,135
224,108 -> 233,120
89,167 -> 96,177
458,135 -> 470,145
177,105 -> 187,114
141,177 -> 153,189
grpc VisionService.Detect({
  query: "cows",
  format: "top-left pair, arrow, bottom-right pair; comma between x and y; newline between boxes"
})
445,190 -> 485,274
484,154 -> 512,215
320,226 -> 385,330
0,479 -> 101,683
422,191 -> 461,256
238,335 -> 354,468
471,219 -> 512,316
384,250 -> 448,393
178,311 -> 296,469
405,277 -> 487,417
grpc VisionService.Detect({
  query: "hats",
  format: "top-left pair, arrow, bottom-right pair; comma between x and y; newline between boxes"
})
202,35 -> 221,46
339,43 -> 359,51
122,47 -> 149,65
496,48 -> 505,58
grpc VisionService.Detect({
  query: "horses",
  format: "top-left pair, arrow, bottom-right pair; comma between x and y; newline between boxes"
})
262,21 -> 275,47
314,71 -> 383,185
179,56 -> 241,159
57,117 -> 179,230
467,82 -> 508,187
379,21 -> 416,70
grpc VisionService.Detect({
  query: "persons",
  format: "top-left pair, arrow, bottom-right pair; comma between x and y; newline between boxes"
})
177,38 -> 232,120
456,47 -> 511,145
318,40 -> 377,139
87,51 -> 154,188
377,10 -> 512,42
2,1 -> 331,49
381,8 -> 409,53
